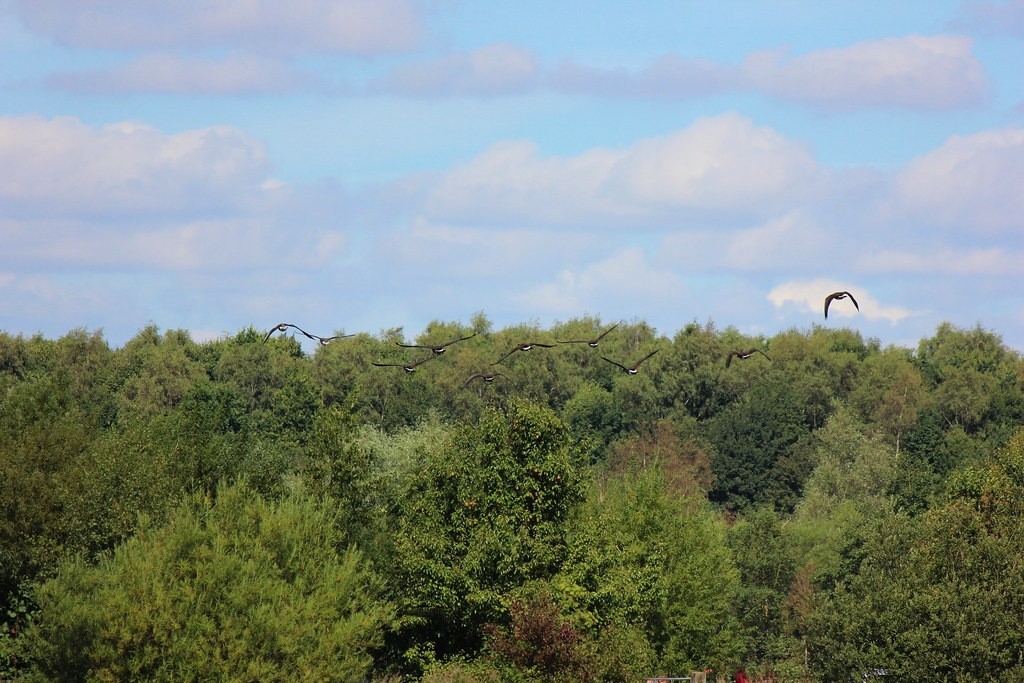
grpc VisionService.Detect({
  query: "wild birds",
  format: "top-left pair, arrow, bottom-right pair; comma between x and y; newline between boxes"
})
600,349 -> 660,376
490,342 -> 558,367
558,321 -> 620,347
294,330 -> 356,347
725,348 -> 772,370
824,291 -> 860,319
460,372 -> 514,389
395,332 -> 477,355
262,323 -> 316,347
369,353 -> 436,373
